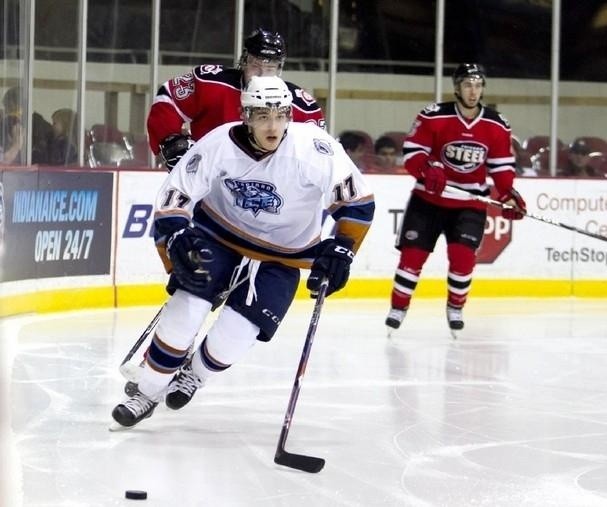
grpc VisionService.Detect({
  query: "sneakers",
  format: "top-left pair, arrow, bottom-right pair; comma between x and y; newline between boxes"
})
383,306 -> 408,338
108,389 -> 159,434
161,350 -> 206,413
124,358 -> 147,397
446,305 -> 465,340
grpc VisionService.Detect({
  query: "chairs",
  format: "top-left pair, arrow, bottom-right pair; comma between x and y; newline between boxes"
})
511,133 -> 607,181
87,123 -> 153,169
356,128 -> 408,169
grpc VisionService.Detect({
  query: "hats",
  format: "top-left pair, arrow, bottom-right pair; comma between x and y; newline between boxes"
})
570,139 -> 591,154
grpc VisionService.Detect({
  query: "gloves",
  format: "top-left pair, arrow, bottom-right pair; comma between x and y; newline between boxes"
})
498,187 -> 527,221
420,156 -> 447,195
305,240 -> 355,299
159,133 -> 196,173
152,208 -> 217,292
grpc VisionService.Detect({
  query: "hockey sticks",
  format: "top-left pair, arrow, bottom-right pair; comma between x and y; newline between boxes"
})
118,303 -> 165,382
274,278 -> 329,473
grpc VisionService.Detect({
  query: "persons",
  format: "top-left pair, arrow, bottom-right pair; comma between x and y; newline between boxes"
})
111,74 -> 375,427
384,63 -> 526,329
556,138 -> 597,177
0,116 -> 23,167
375,137 -> 398,167
510,136 -> 538,176
145,26 -> 328,172
0,87 -> 51,140
33,108 -> 79,167
338,131 -> 365,164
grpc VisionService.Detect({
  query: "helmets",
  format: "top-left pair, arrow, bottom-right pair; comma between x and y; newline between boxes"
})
240,25 -> 288,64
239,75 -> 294,116
453,61 -> 486,88
